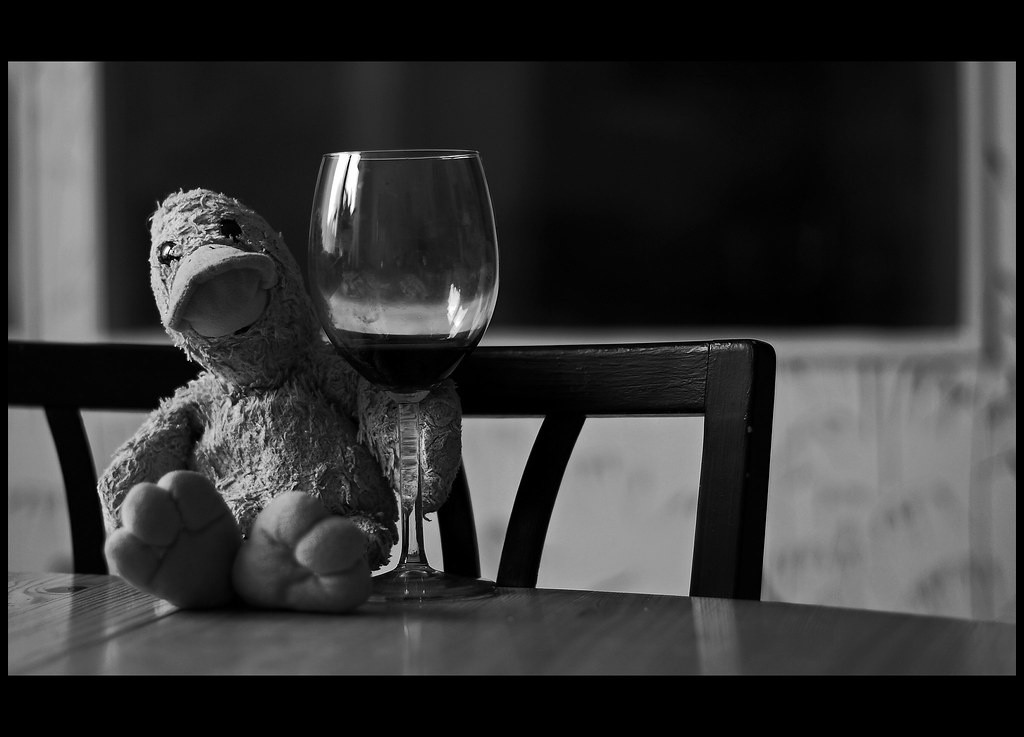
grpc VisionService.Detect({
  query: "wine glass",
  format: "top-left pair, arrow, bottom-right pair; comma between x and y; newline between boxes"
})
306,147 -> 501,599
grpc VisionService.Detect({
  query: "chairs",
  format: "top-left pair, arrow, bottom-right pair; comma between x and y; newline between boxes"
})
0,337 -> 779,604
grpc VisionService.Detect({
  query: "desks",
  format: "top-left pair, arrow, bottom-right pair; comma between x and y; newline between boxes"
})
8,567 -> 1016,676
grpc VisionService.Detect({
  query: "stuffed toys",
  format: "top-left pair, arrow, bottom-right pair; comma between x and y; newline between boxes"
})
95,188 -> 461,611
